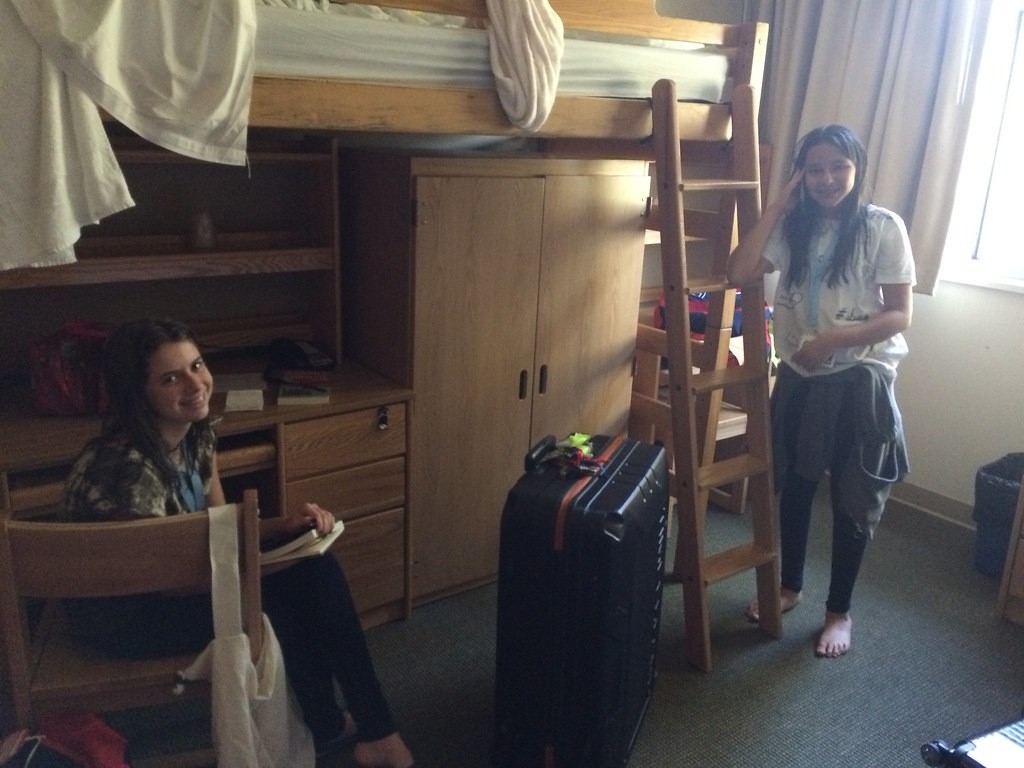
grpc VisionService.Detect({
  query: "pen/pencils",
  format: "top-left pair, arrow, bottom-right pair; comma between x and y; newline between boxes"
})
270,377 -> 328,394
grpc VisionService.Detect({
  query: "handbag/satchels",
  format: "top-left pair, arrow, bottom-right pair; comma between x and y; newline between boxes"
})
179,504 -> 316,768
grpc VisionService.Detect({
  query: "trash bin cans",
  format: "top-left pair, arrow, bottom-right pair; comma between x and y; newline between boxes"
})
972,453 -> 1024,579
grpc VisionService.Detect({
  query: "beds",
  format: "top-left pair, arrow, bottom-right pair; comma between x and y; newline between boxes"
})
0,0 -> 781,581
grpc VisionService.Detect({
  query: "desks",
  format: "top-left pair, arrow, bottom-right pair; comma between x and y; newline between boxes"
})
0,340 -> 417,639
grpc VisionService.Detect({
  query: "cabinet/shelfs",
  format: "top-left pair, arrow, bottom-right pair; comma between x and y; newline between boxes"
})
337,143 -> 652,621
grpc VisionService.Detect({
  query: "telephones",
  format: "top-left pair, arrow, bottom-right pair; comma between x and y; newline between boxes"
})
269,335 -> 334,370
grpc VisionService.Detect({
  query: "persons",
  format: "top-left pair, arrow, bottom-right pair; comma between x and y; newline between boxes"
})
51,315 -> 419,767
724,124 -> 917,657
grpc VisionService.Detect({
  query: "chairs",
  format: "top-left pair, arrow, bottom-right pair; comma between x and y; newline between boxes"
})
0,471 -> 318,768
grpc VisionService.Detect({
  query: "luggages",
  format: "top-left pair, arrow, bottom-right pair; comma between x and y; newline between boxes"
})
496,430 -> 669,768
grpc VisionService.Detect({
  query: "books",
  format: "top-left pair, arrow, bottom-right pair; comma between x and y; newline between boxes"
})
258,519 -> 345,566
277,383 -> 330,405
